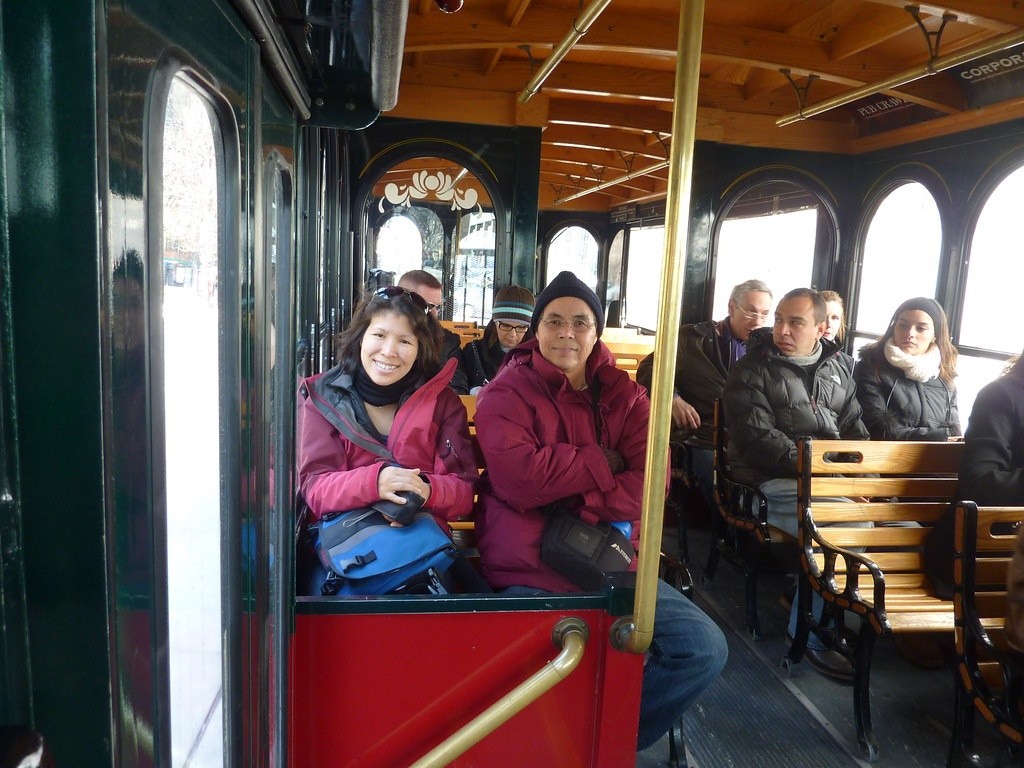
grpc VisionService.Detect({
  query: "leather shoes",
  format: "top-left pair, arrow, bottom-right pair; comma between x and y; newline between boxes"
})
785,630 -> 857,680
898,632 -> 945,666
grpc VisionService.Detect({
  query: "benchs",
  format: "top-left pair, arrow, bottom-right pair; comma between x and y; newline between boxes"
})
442,321 -> 485,530
601,329 -> 1024,768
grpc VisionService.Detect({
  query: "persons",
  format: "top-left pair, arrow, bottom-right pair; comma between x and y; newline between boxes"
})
399,270 -> 534,395
725,288 -> 963,684
636,280 -> 845,470
475,270 -> 730,753
921,350 -> 1024,598
1004,522 -> 1024,648
297,285 -> 479,593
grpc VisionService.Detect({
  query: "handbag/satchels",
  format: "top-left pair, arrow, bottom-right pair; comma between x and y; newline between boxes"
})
664,491 -> 686,525
541,504 -> 610,583
579,531 -> 634,591
658,552 -> 693,602
307,507 -> 461,595
922,493 -> 956,601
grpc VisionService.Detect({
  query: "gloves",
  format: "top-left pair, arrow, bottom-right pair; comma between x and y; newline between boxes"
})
604,449 -> 627,472
552,493 -> 585,505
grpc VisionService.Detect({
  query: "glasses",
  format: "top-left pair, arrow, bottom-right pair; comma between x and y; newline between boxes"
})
734,299 -> 770,322
538,317 -> 596,334
497,322 -> 528,333
374,286 -> 414,302
427,301 -> 443,313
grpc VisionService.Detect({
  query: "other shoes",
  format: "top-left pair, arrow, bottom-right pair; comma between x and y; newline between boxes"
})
781,593 -> 793,612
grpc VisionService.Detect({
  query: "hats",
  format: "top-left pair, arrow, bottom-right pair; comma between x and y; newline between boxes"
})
492,285 -> 535,327
531,271 -> 605,338
